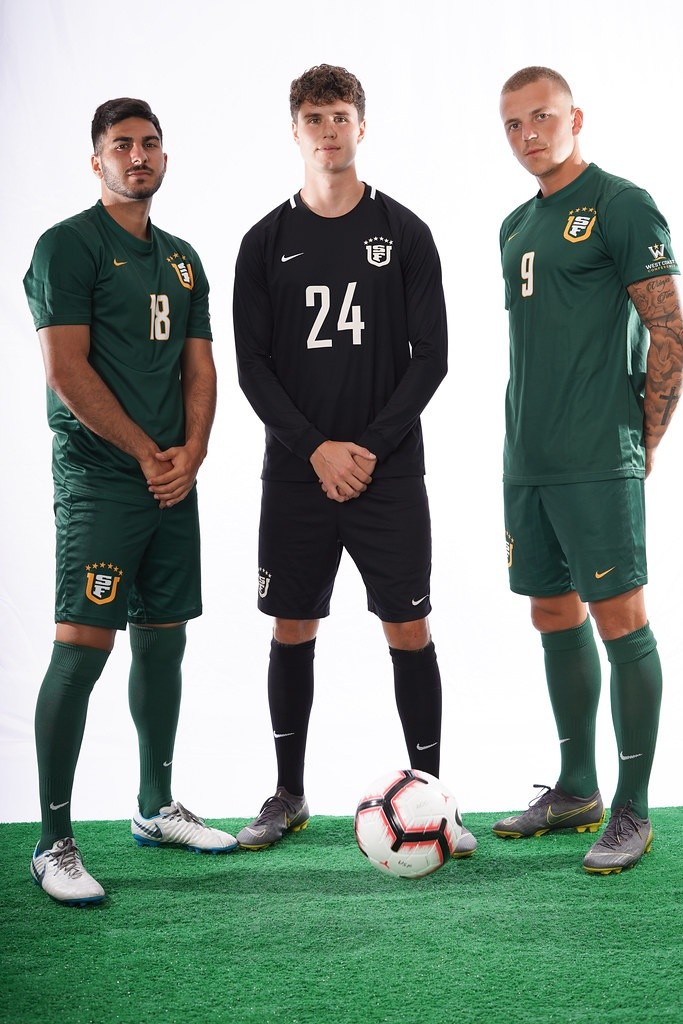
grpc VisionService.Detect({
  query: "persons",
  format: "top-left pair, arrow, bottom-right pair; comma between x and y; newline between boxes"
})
492,64 -> 683,878
21,96 -> 241,905
231,64 -> 478,864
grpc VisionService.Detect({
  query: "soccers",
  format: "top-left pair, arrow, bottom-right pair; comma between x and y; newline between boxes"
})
352,767 -> 464,878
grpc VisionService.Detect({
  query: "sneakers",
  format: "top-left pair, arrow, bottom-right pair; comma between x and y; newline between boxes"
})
491,782 -> 606,839
583,806 -> 653,874
30,836 -> 106,908
236,787 -> 309,851
449,825 -> 478,859
130,800 -> 239,854
